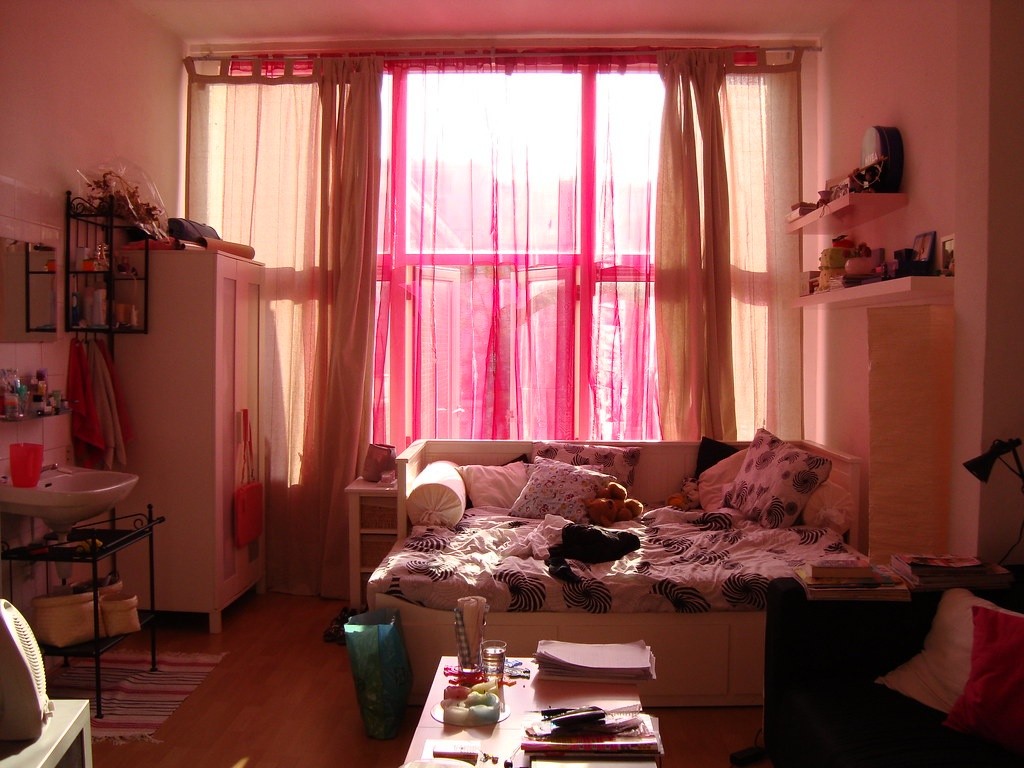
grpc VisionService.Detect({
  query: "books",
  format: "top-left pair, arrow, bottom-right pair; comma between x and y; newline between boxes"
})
793,554 -> 1017,600
521,712 -> 664,756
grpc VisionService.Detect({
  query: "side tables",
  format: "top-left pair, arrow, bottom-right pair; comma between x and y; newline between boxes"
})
345,476 -> 402,615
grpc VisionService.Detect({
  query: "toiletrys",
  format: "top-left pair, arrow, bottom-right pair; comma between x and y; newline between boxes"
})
0,367 -> 71,418
71,286 -> 138,328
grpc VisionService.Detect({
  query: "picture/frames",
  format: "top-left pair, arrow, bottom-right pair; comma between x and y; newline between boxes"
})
939,234 -> 956,275
910,230 -> 936,262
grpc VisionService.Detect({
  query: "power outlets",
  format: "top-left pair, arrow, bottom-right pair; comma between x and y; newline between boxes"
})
66,446 -> 72,464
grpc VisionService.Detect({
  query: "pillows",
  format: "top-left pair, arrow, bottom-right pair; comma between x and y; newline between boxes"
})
874,588 -> 1024,746
680,429 -> 833,529
406,441 -> 643,525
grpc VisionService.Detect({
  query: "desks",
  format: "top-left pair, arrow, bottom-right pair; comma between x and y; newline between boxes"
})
0,699 -> 94,768
402,654 -> 660,768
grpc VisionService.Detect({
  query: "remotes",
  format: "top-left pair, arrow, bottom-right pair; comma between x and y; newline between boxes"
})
541,707 -> 606,727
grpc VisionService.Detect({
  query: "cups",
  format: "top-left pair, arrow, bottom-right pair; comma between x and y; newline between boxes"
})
9,442 -> 44,488
480,640 -> 507,683
116,303 -> 130,323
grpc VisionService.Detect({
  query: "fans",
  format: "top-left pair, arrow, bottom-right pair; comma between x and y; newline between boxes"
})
0,598 -> 57,741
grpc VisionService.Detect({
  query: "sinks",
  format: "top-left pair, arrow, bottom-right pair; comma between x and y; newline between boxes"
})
0,462 -> 141,534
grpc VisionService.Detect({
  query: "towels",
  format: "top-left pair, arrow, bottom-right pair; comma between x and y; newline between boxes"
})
64,337 -> 137,474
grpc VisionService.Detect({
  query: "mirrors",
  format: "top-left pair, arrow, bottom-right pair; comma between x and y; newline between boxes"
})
0,237 -> 61,347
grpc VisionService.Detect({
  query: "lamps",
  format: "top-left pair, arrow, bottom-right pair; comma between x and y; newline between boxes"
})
961,437 -> 1024,510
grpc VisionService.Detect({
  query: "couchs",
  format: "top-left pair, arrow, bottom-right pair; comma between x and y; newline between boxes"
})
764,575 -> 1024,768
365,438 -> 860,709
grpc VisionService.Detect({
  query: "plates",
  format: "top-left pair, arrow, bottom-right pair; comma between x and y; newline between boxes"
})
431,702 -> 511,727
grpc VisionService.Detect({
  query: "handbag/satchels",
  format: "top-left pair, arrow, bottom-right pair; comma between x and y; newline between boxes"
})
343,607 -> 412,741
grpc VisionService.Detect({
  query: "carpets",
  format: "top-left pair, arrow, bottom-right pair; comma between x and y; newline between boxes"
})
40,650 -> 230,744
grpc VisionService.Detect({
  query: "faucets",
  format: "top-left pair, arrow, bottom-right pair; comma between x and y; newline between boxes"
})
41,462 -> 60,474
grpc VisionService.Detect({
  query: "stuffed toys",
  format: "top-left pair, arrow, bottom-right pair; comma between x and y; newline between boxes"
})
585,481 -> 644,528
561,523 -> 641,565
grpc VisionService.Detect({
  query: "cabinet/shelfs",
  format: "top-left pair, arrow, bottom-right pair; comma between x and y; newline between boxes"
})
114,248 -> 272,633
784,190 -> 956,307
25,242 -> 59,332
64,190 -> 152,335
10,504 -> 167,716
